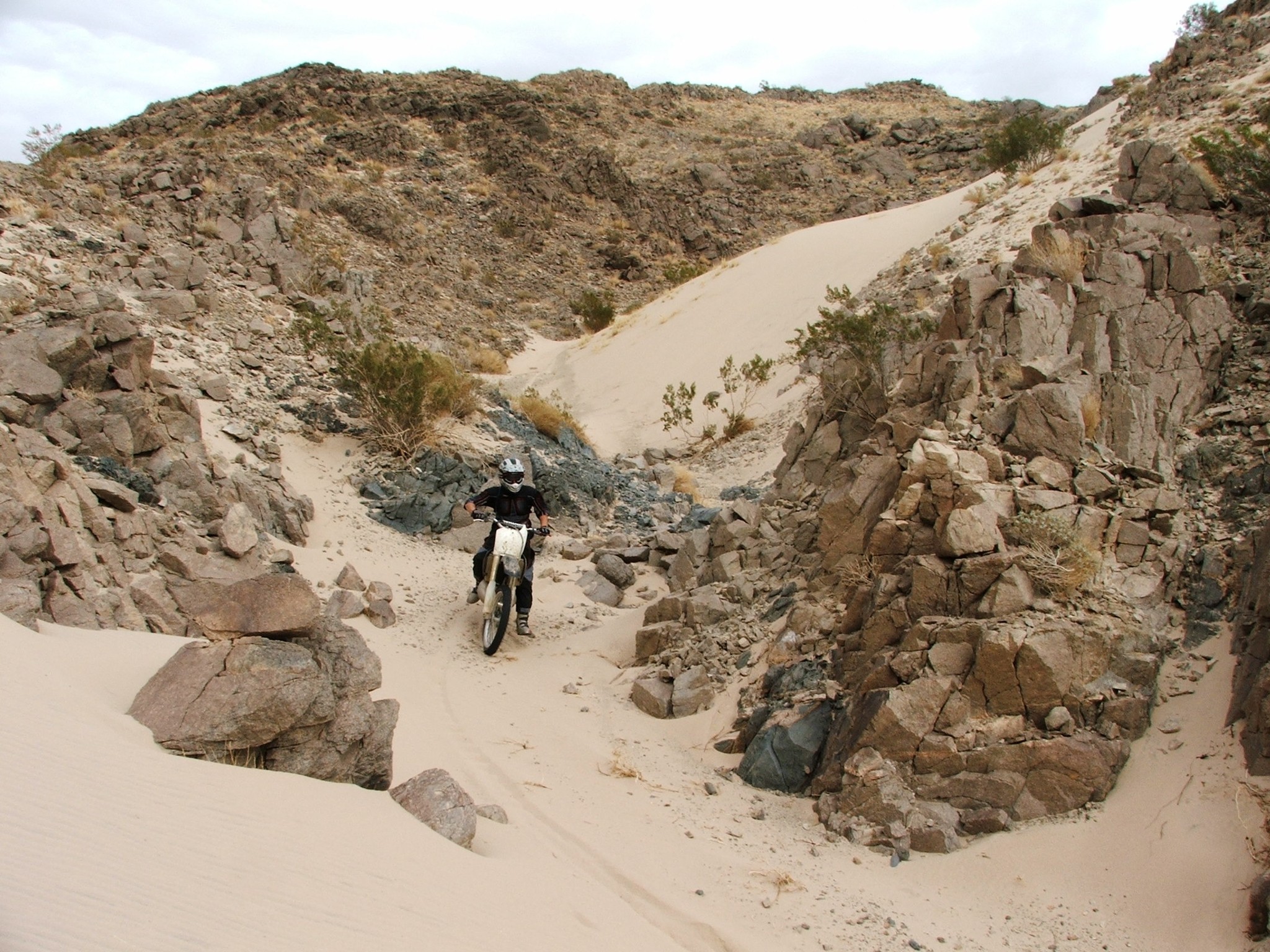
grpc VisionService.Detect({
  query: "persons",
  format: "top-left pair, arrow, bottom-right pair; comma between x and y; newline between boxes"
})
464,458 -> 550,635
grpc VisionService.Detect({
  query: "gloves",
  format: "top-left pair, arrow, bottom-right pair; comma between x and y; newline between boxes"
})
537,525 -> 550,536
471,508 -> 485,522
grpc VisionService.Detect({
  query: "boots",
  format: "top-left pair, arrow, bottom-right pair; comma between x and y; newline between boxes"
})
517,613 -> 532,635
467,582 -> 481,603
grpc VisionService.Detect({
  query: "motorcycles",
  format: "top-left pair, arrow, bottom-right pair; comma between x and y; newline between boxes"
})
476,511 -> 552,657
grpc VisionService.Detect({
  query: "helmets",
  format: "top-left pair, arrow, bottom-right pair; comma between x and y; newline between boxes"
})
498,458 -> 526,492
